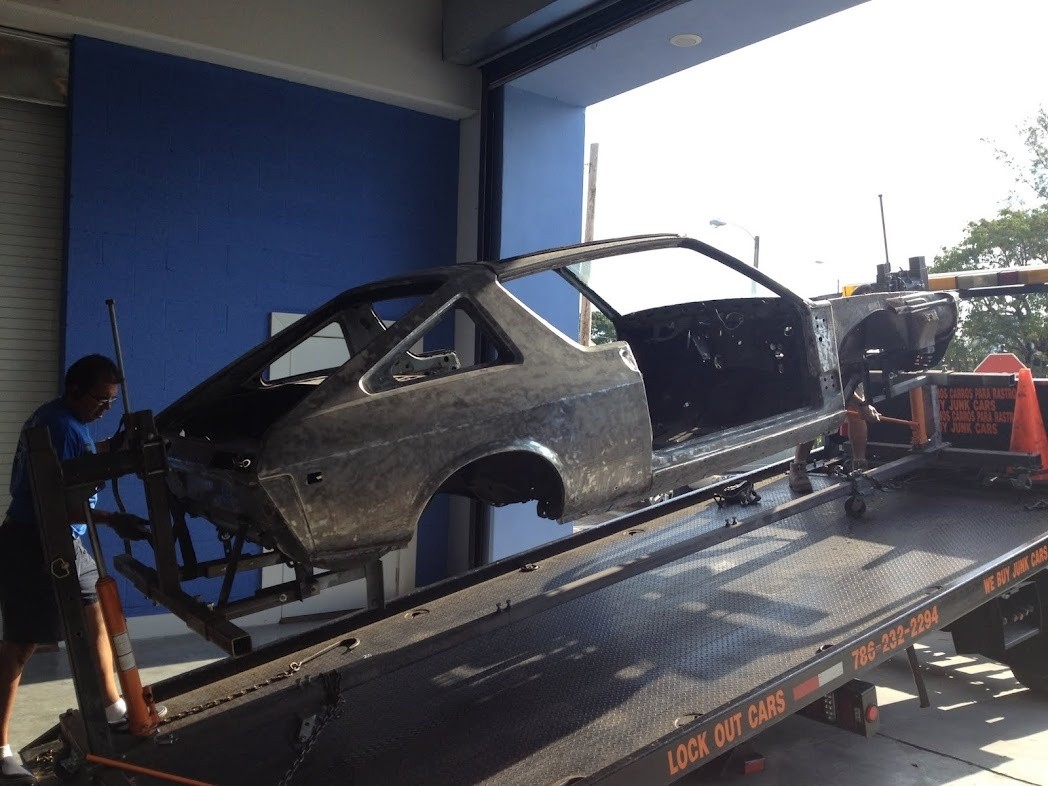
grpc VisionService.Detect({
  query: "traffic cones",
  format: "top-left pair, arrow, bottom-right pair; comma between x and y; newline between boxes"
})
1008,368 -> 1047,487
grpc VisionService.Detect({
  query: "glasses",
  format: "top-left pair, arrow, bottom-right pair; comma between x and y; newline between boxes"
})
80,385 -> 117,406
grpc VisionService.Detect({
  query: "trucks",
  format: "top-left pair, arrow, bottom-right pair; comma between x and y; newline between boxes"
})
17,264 -> 1047,786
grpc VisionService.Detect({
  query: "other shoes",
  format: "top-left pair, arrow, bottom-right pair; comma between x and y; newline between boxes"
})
109,705 -> 167,735
0,757 -> 32,783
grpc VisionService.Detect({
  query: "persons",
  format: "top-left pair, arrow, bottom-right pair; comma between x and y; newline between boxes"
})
788,284 -> 883,491
0,355 -> 168,786
